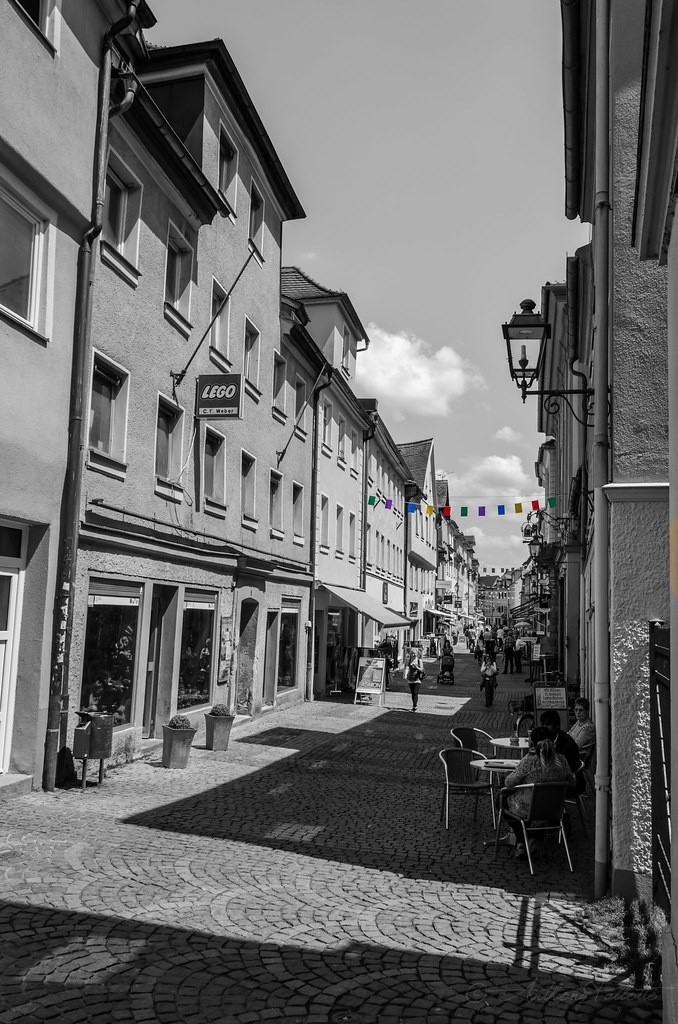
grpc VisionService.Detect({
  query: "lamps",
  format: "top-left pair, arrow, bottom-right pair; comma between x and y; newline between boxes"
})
502,297 -> 612,428
529,524 -> 582,565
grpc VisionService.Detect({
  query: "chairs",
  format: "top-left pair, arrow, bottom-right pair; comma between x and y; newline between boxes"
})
438,726 -> 596,876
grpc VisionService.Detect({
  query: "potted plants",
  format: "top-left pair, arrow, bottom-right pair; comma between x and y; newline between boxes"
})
204,704 -> 235,751
161,714 -> 198,769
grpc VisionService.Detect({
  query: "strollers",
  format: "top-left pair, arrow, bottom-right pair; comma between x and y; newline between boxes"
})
437,653 -> 455,684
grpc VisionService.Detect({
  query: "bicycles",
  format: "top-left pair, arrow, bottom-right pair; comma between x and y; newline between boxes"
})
508,653 -> 576,737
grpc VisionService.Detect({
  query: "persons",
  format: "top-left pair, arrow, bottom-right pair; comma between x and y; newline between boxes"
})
452,623 -> 540,674
181,638 -> 212,695
565,698 -> 595,748
480,654 -> 496,707
541,711 -> 583,789
441,640 -> 454,658
404,649 -> 423,711
505,727 -> 572,859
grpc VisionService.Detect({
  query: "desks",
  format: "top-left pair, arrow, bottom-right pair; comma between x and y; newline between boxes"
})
492,737 -> 529,759
470,758 -> 524,828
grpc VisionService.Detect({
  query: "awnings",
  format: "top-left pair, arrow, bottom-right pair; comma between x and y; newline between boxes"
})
322,582 -> 412,633
427,610 -> 454,617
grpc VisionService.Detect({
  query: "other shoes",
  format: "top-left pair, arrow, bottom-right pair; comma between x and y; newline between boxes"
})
515,840 -> 539,857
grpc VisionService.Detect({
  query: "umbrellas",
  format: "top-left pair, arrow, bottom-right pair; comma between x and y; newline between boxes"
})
514,621 -> 531,635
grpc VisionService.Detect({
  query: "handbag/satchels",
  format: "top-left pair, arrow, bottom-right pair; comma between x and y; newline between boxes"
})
418,659 -> 428,680
